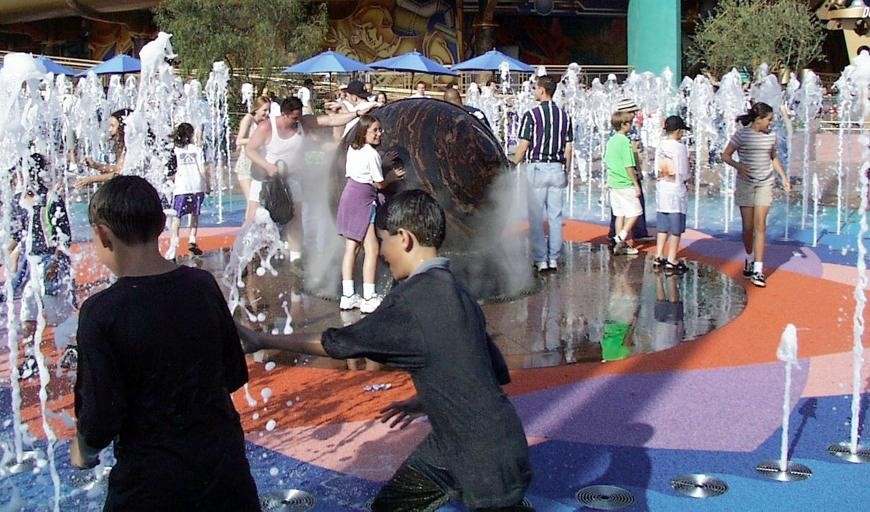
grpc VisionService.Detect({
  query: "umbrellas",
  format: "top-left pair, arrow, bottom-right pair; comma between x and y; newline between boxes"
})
1,52 -> 80,78
279,46 -> 377,102
364,47 -> 462,96
77,53 -> 154,74
450,45 -> 537,84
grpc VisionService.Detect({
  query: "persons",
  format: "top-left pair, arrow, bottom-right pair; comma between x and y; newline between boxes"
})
230,185 -> 533,512
513,76 -> 575,271
72,173 -> 264,512
2,76 -> 470,386
605,101 -> 841,287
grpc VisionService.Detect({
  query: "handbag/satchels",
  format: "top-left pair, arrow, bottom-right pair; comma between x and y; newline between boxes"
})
167,154 -> 176,175
258,173 -> 295,226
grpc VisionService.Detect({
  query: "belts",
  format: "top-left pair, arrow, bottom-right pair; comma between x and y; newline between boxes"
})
531,159 -> 561,162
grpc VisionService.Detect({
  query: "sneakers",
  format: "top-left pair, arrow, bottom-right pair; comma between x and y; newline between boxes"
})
751,272 -> 767,287
359,294 -> 383,314
289,258 -> 306,279
742,259 -> 755,280
339,293 -> 367,311
608,231 -> 655,256
533,260 -> 557,273
18,357 -> 40,380
57,345 -> 78,369
188,243 -> 203,255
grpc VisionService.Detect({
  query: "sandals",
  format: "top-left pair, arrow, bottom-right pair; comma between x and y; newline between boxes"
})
653,256 -> 666,267
664,260 -> 689,273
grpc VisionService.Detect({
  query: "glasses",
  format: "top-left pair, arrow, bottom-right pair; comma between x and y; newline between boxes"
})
367,129 -> 383,133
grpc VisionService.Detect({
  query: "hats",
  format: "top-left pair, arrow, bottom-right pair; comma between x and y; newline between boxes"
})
664,115 -> 691,132
346,80 -> 372,99
617,98 -> 640,113
